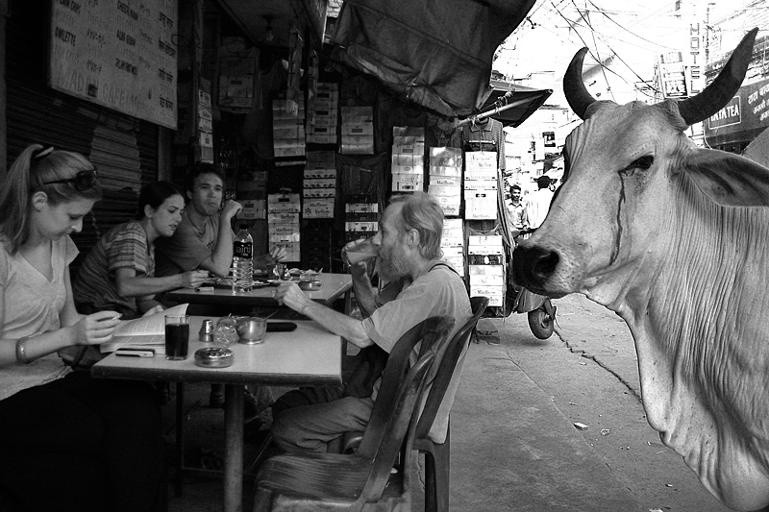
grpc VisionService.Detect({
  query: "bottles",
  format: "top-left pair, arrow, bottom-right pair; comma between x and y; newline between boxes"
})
198,315 -> 239,345
229,224 -> 254,292
264,264 -> 301,282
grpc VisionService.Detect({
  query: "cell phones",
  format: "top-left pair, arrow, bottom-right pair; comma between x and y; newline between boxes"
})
117,348 -> 155,357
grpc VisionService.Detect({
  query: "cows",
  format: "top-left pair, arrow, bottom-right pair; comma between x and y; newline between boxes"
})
505,24 -> 769,512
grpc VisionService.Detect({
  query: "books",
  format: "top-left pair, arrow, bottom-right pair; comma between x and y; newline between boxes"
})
98,302 -> 190,354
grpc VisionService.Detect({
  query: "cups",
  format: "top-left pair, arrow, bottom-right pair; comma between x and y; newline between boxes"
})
343,235 -> 378,265
164,312 -> 192,362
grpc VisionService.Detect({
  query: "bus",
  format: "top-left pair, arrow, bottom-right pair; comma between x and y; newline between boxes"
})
507,225 -> 558,339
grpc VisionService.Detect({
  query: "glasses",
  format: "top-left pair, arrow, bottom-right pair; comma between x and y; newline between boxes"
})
42,169 -> 97,190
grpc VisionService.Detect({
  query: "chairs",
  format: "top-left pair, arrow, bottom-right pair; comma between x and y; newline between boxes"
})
253,314 -> 458,511
330,297 -> 491,511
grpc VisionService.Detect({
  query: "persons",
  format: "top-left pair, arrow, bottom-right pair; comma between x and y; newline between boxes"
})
504,184 -> 531,233
70,181 -> 209,320
158,162 -> 244,280
0,143 -> 160,512
271,191 -> 475,461
527,176 -> 555,229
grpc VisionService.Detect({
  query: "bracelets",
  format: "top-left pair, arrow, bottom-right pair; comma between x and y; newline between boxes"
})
16,337 -> 31,365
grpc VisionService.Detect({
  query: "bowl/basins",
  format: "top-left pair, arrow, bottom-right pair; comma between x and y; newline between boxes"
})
235,317 -> 267,346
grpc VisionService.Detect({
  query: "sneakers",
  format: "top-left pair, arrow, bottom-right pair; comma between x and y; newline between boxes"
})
209,391 -> 225,408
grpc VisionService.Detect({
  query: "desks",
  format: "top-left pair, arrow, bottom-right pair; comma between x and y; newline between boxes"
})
165,270 -> 356,357
90,313 -> 346,510
